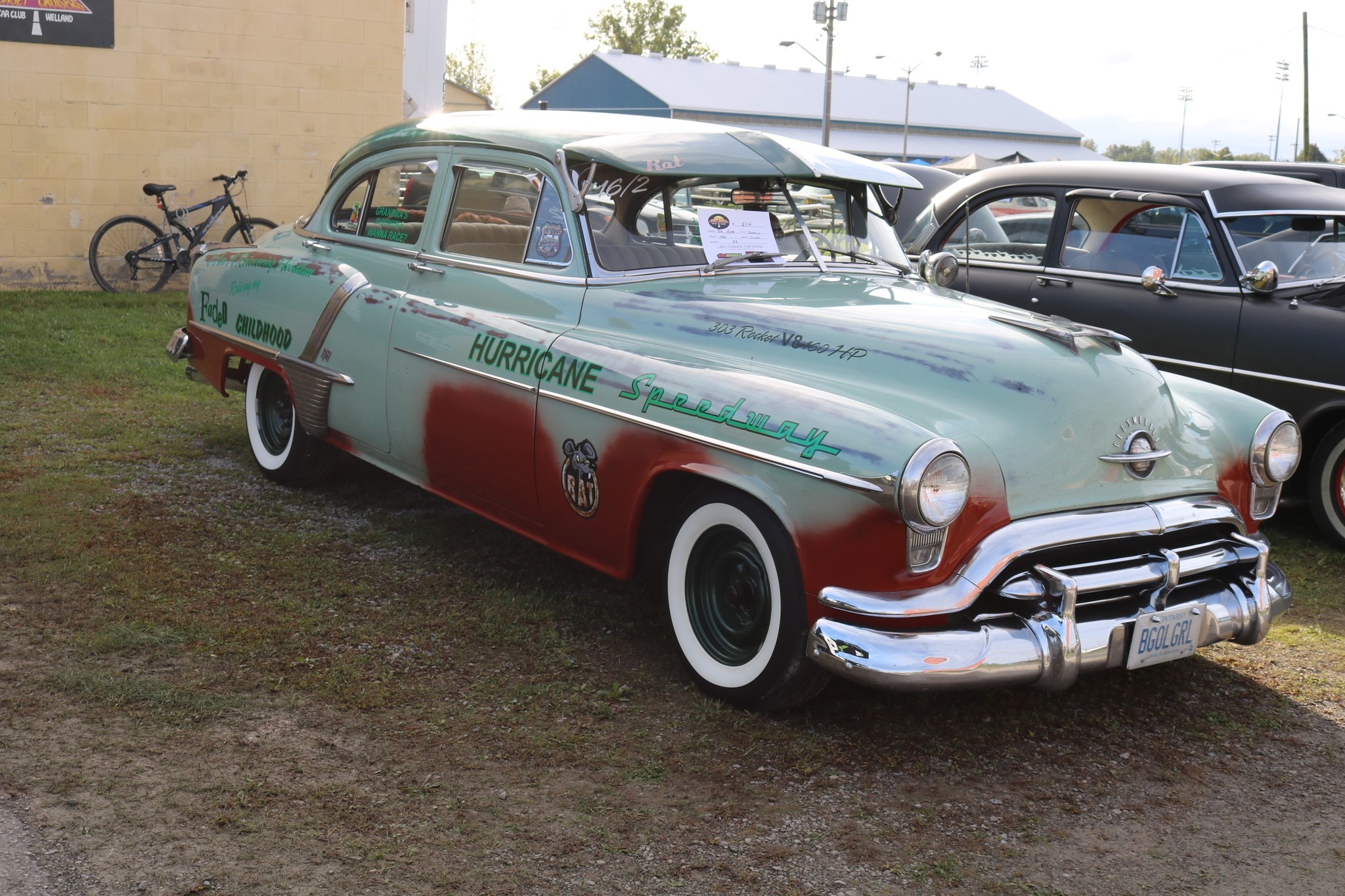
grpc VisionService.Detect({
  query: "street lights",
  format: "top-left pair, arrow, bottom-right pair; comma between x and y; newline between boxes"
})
1274,58 -> 1289,163
779,41 -> 832,147
875,51 -> 942,164
970,54 -> 989,87
1177,85 -> 1193,166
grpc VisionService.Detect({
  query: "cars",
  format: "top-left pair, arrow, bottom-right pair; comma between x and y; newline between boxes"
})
399,160 -> 1345,550
163,110 -> 1300,717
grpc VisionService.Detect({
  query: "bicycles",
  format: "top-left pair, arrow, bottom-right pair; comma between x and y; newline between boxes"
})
89,171 -> 282,296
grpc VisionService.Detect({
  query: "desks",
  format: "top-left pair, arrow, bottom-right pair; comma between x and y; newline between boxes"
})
583,187 -> 845,255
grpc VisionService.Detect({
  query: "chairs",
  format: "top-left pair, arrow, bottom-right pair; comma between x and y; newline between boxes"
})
1160,253 -> 1219,277
1071,252 -> 1169,278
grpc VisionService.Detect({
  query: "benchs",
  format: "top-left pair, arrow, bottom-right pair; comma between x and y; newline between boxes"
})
365,222 -> 709,270
